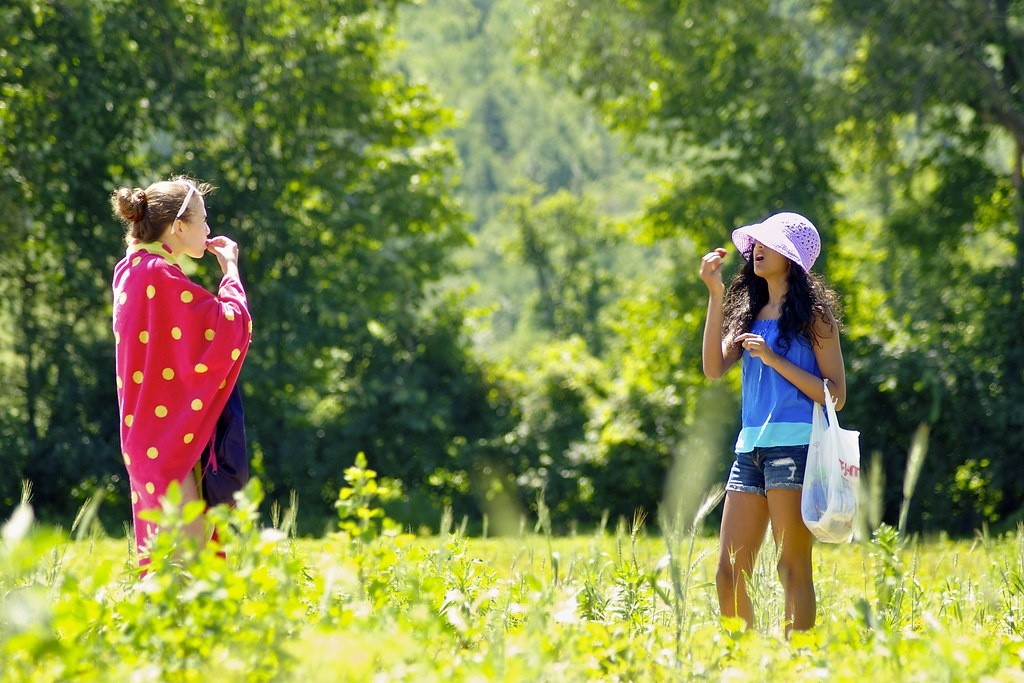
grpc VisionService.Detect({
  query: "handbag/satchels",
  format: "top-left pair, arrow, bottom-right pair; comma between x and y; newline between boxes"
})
800,376 -> 861,545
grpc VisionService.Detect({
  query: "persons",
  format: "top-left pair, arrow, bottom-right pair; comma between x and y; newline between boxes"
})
110,178 -> 252,578
697,213 -> 847,640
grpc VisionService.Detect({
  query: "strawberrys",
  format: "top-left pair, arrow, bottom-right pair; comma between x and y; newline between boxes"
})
715,247 -> 727,258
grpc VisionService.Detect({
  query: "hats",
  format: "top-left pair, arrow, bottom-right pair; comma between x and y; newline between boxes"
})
732,211 -> 820,275
